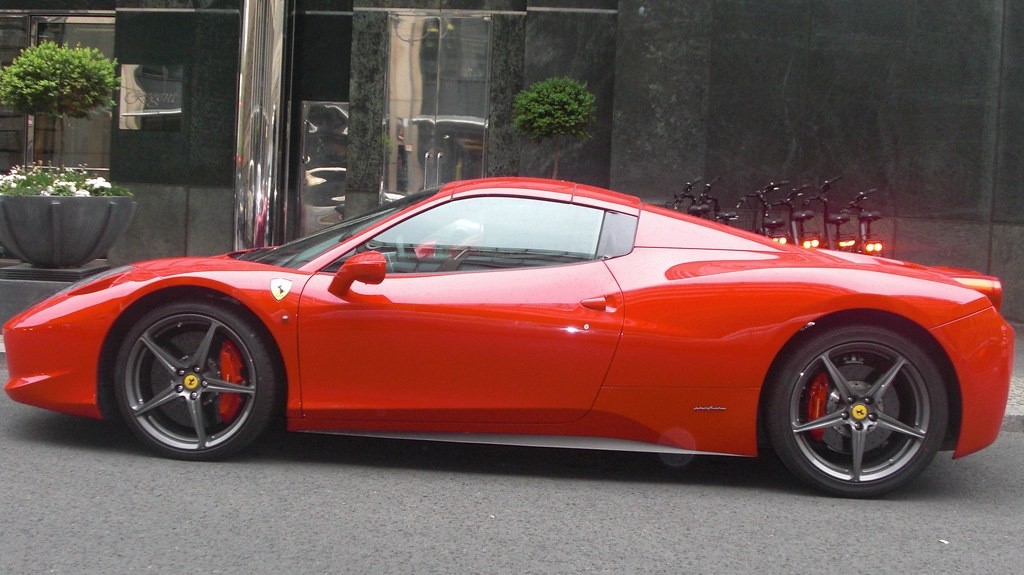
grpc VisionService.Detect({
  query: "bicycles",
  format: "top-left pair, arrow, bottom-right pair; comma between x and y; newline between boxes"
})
800,176 -> 856,254
736,181 -> 793,245
838,189 -> 883,258
762,186 -> 822,249
697,177 -> 739,225
665,177 -> 711,221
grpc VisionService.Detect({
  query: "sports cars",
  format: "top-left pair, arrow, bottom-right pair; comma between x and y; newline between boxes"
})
2,178 -> 1015,501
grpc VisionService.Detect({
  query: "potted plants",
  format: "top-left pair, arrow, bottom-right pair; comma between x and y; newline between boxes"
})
0,42 -> 137,269
